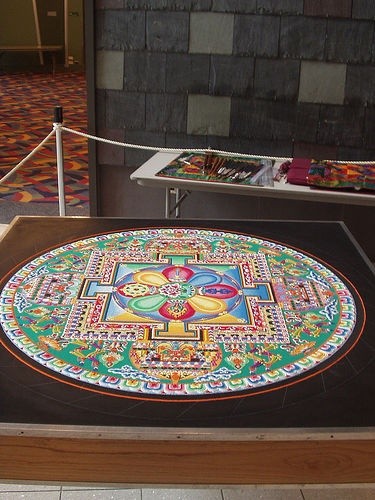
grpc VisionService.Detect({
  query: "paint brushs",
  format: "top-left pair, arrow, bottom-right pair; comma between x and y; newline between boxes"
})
183,153 -> 264,182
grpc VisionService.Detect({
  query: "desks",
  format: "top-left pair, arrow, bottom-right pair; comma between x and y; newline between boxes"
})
129,150 -> 375,218
0,214 -> 374,484
